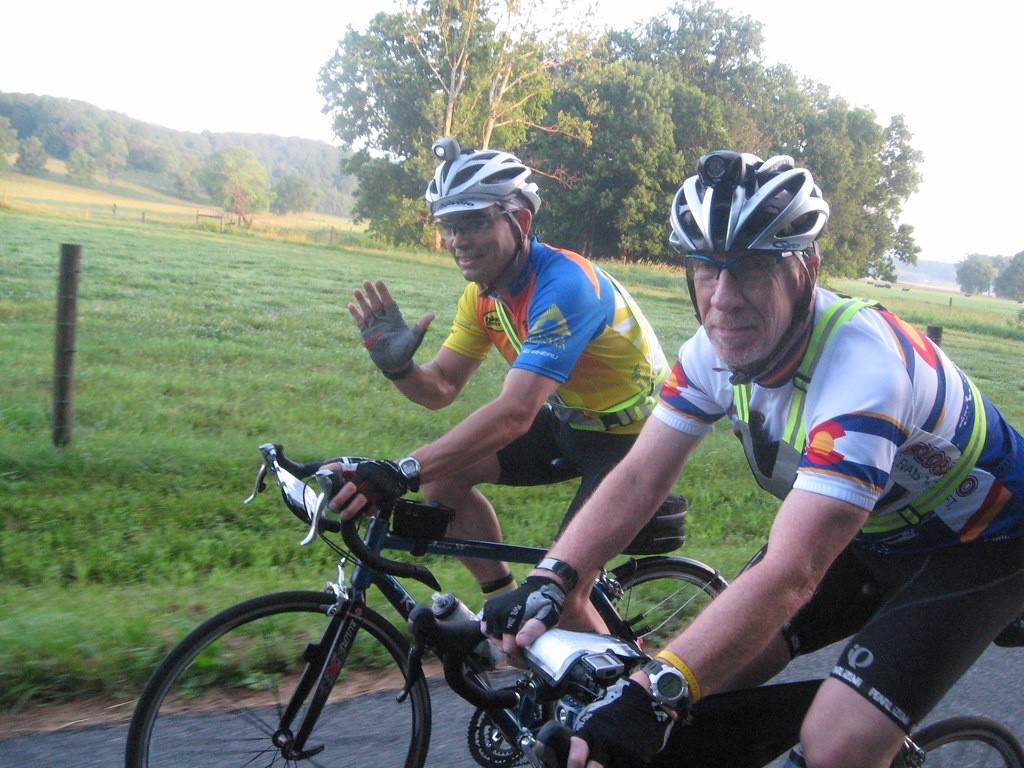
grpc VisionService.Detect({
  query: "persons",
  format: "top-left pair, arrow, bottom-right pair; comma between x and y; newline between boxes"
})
330,139 -> 672,663
481,150 -> 1024,768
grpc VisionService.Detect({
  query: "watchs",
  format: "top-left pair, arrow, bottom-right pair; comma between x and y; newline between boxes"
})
400,456 -> 420,492
641,657 -> 690,722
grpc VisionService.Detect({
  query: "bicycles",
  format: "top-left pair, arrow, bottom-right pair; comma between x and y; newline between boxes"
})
122,443 -> 731,767
394,580 -> 1023,766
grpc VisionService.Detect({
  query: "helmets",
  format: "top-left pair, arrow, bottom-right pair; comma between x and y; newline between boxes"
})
426,135 -> 542,218
668,149 -> 831,251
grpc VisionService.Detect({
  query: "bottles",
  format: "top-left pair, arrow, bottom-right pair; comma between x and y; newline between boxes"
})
430,591 -> 502,668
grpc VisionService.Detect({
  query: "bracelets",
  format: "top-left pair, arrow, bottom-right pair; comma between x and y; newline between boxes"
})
655,650 -> 700,704
534,558 -> 579,590
382,358 -> 414,381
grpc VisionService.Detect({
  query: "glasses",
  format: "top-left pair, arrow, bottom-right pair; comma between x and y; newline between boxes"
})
433,209 -> 520,237
682,251 -> 810,283
